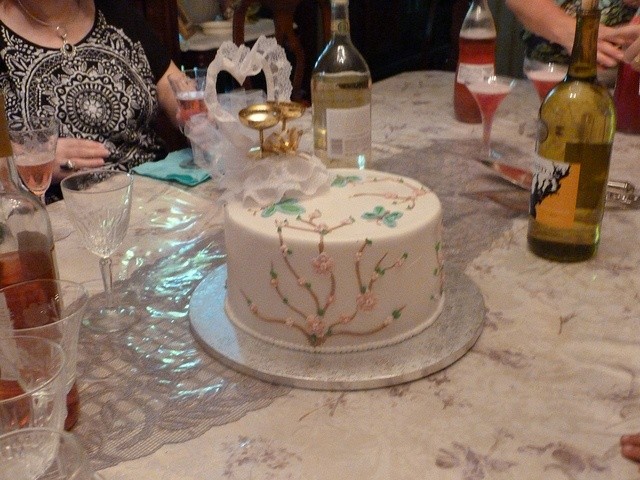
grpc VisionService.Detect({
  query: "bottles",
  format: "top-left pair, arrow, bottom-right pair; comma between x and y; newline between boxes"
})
0,88 -> 80,433
453,3 -> 497,120
527,2 -> 616,265
310,5 -> 374,167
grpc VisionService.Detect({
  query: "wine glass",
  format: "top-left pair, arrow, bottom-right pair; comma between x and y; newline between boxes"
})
0,335 -> 65,480
461,70 -> 515,164
167,68 -> 215,170
61,168 -> 144,332
9,116 -> 57,207
0,279 -> 90,480
524,62 -> 574,109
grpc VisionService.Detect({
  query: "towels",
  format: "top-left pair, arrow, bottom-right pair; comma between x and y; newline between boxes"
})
129,145 -> 215,186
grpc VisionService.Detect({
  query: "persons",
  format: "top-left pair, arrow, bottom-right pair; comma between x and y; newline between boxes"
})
504,0 -> 640,84
620,432 -> 640,463
0,0 -> 208,204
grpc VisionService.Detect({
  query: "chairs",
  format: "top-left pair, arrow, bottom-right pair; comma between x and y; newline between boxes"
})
233,0 -> 333,107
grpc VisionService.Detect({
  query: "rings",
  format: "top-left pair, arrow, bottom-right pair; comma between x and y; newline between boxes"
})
66,159 -> 73,169
634,56 -> 640,65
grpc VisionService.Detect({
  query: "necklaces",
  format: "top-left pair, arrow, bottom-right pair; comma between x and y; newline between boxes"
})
18,1 -> 80,58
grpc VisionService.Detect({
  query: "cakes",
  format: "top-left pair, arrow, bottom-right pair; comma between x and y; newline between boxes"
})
221,167 -> 445,352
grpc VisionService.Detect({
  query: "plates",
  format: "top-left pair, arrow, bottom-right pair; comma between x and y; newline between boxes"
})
201,20 -> 233,35
